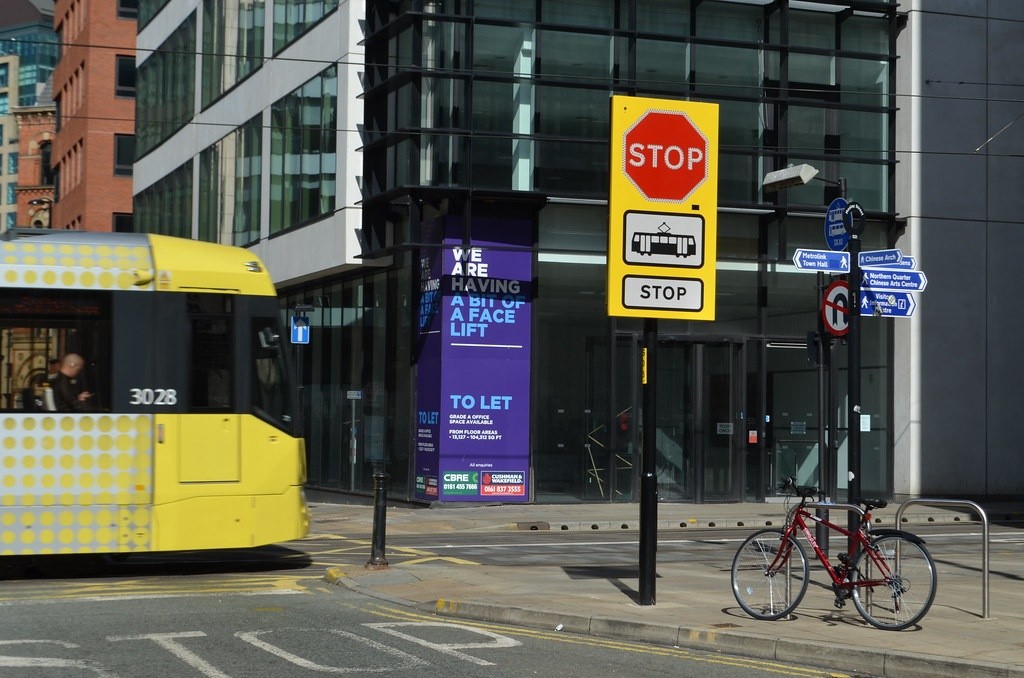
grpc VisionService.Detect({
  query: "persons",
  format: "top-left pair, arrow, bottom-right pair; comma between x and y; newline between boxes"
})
47,353 -> 94,414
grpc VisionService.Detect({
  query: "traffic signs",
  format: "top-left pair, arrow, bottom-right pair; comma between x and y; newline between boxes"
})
859,291 -> 916,318
793,249 -> 850,274
857,250 -> 902,267
901,256 -> 916,270
860,268 -> 928,290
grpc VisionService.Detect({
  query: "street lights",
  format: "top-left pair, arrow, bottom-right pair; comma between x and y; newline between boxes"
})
760,161 -> 865,599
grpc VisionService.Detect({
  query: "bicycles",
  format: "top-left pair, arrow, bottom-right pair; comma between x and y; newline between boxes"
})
728,477 -> 938,631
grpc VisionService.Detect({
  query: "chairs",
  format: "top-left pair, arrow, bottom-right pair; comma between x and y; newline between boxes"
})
35,387 -> 57,411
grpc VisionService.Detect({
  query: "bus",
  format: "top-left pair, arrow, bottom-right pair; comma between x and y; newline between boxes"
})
0,226 -> 313,573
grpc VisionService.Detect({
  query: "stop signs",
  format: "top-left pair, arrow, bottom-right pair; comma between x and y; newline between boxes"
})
623,110 -> 709,202
